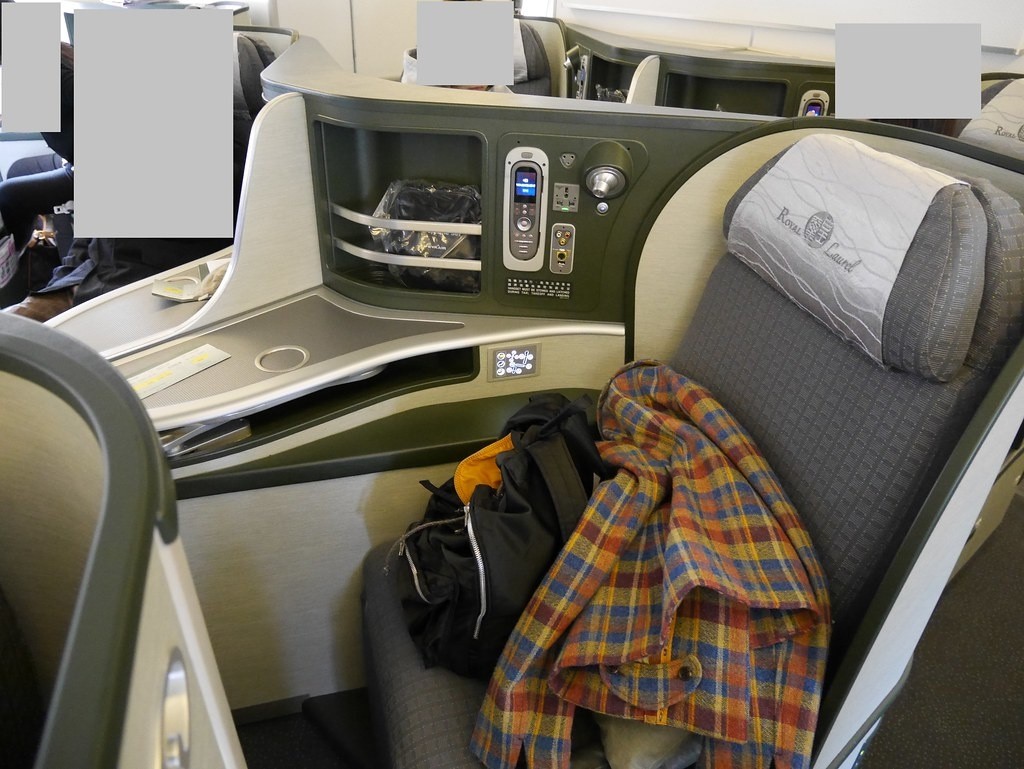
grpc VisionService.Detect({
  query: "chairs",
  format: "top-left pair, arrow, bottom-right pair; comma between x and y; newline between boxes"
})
233,23 -> 299,248
954,72 -> 1024,161
400,14 -> 569,102
351,114 -> 1024,769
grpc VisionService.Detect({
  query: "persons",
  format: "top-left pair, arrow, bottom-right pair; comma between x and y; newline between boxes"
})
0,236 -> 185,323
0,41 -> 75,245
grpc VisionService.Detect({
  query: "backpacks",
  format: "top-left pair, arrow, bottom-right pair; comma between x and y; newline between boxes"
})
396,391 -> 612,681
